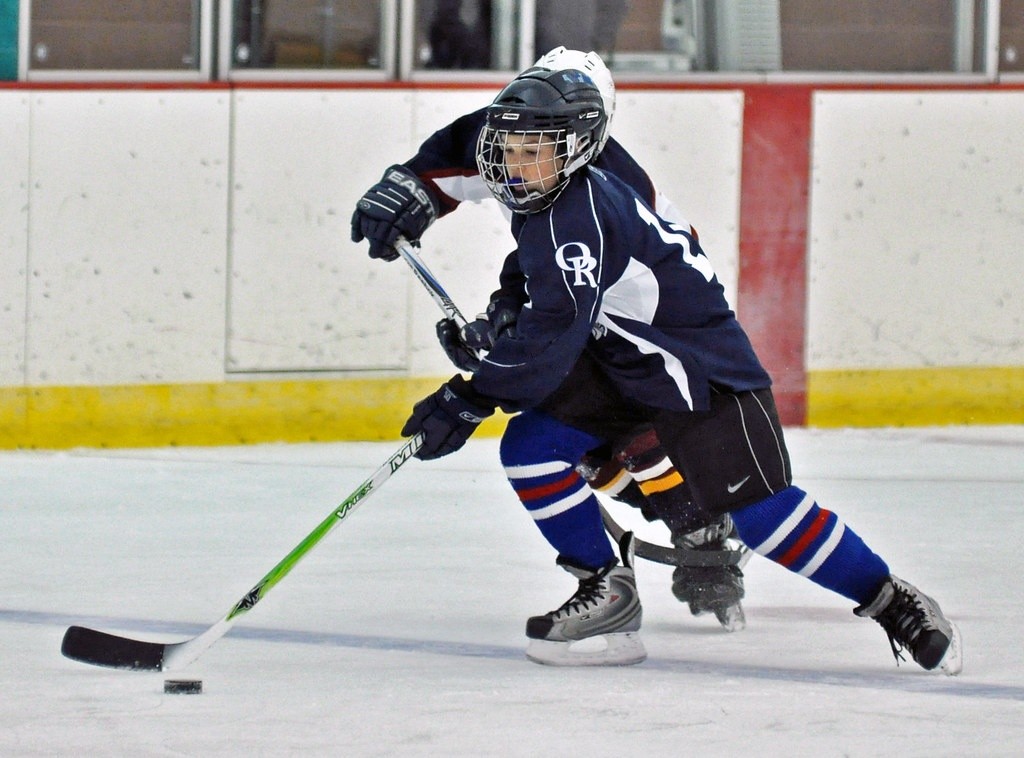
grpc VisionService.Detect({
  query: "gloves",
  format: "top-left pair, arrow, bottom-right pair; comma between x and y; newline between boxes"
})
435,313 -> 492,373
351,164 -> 440,262
400,373 -> 495,461
486,298 -> 521,339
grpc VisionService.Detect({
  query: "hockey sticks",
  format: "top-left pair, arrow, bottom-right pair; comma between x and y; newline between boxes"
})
394,235 -> 745,571
55,428 -> 429,676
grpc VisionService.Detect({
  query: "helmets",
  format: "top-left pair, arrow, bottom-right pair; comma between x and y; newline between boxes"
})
533,45 -> 616,163
475,66 -> 606,215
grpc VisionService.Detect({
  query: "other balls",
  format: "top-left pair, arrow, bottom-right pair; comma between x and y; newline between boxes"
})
163,679 -> 204,695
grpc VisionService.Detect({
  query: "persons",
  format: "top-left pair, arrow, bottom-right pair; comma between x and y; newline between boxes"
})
398,67 -> 962,678
348,46 -> 747,634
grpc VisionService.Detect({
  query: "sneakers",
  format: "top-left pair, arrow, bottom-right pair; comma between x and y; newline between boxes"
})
525,530 -> 647,666
670,517 -> 746,632
853,573 -> 963,676
